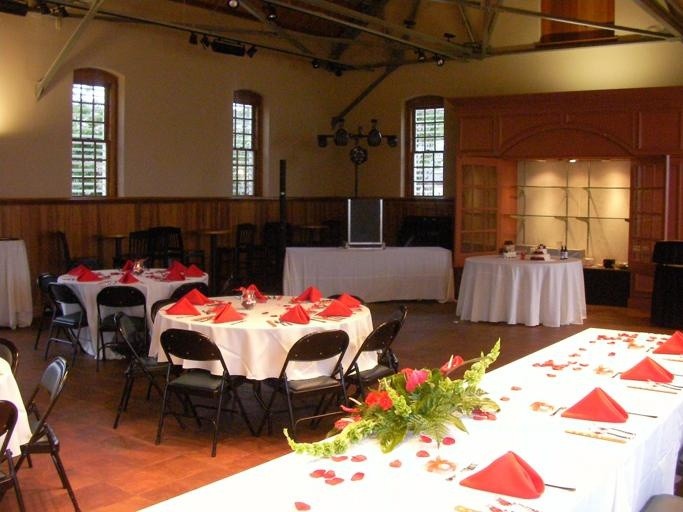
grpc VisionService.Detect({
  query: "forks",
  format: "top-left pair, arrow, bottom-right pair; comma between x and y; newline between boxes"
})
443,461 -> 478,483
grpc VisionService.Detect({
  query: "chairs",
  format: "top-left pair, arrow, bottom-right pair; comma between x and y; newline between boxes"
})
112,311 -> 201,431
8,354 -> 80,511
255,330 -> 349,443
33,274 -> 60,348
0,222 -> 457,327
44,283 -> 88,364
96,287 -> 148,374
152,328 -> 250,458
0,337 -> 19,373
0,401 -> 26,511
315,304 -> 406,428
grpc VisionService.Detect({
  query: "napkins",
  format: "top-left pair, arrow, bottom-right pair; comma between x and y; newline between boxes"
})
165,297 -> 201,315
652,331 -> 682,354
296,286 -> 322,303
561,386 -> 628,423
279,304 -> 310,324
460,451 -> 545,498
213,303 -> 242,323
338,293 -> 361,307
621,356 -> 674,383
316,300 -> 352,317
176,289 -> 211,305
242,285 -> 266,305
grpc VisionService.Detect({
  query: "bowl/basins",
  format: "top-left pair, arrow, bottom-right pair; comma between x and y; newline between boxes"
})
603,259 -> 616,269
615,264 -> 628,270
584,257 -> 594,266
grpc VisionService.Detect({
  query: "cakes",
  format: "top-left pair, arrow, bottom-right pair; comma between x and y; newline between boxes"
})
531,244 -> 552,261
501,241 -> 516,257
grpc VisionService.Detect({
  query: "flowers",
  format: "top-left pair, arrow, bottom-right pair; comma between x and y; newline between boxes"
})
283,336 -> 502,453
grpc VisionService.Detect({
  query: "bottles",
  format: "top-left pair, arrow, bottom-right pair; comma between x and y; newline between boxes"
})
367,118 -> 382,147
560,245 -> 569,260
240,289 -> 257,309
335,118 -> 349,147
133,262 -> 145,275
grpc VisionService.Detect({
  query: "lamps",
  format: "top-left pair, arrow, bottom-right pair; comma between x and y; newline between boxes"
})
35,0 -> 456,77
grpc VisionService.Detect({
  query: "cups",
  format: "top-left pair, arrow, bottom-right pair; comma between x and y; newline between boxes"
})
521,251 -> 525,260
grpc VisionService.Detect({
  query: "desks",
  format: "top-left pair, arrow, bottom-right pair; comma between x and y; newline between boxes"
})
148,289 -> 379,380
0,353 -> 34,456
456,254 -> 587,329
134,327 -> 682,512
